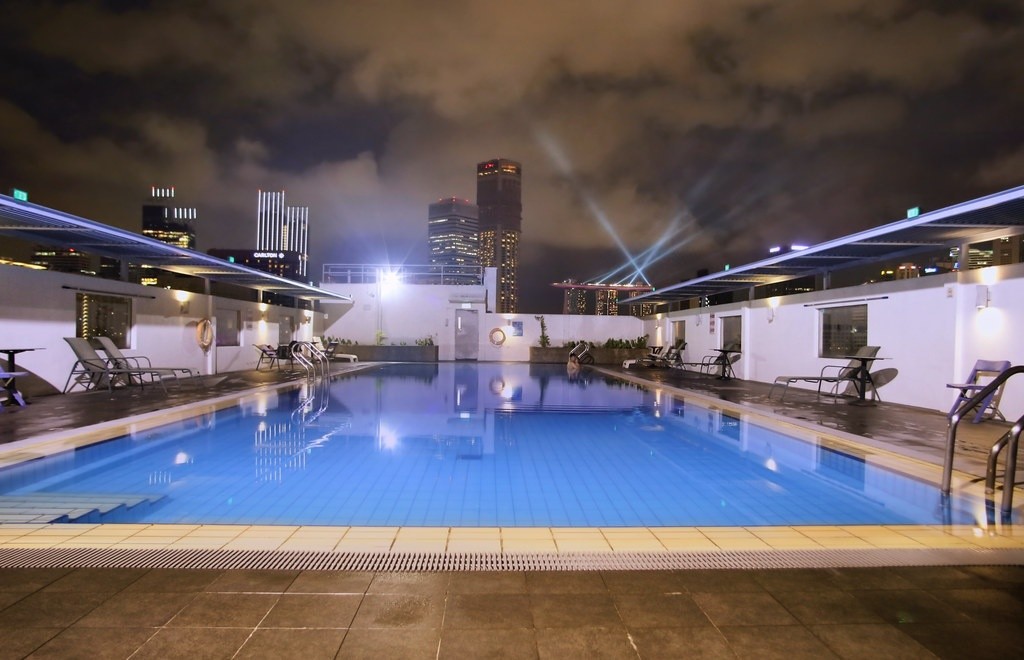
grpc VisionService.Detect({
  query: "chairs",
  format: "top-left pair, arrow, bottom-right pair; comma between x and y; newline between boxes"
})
766,346 -> 882,404
61,337 -> 181,393
252,343 -> 304,371
946,360 -> 1010,424
309,342 -> 338,362
93,336 -> 194,387
623,350 -> 663,369
0,366 -> 30,413
313,336 -> 358,363
635,343 -> 687,370
672,344 -> 737,378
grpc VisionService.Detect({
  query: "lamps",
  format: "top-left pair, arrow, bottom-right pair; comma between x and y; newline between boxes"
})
695,314 -> 701,326
179,301 -> 189,313
766,307 -> 774,320
655,320 -> 658,328
306,317 -> 311,325
260,310 -> 264,320
976,284 -> 990,309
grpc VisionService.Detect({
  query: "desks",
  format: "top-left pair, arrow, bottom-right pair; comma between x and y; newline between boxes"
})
647,346 -> 662,368
0,348 -> 46,406
842,356 -> 892,407
709,349 -> 741,381
